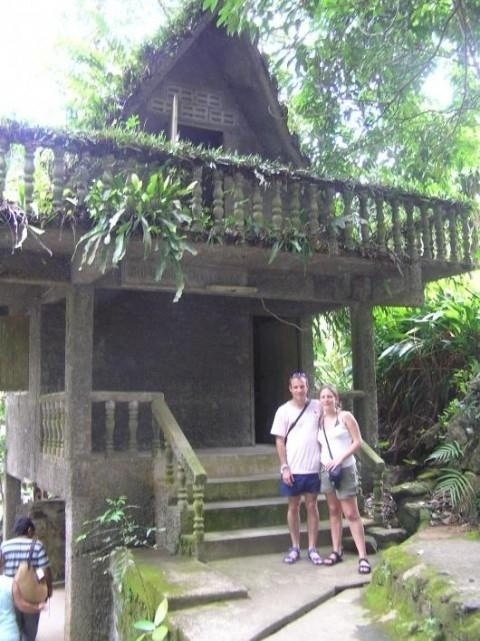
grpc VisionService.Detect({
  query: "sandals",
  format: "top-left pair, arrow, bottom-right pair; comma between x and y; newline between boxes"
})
307,548 -> 323,565
324,549 -> 344,565
283,547 -> 301,564
358,558 -> 371,573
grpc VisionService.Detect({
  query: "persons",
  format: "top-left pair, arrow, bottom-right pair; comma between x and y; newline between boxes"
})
269,368 -> 371,574
0,515 -> 53,641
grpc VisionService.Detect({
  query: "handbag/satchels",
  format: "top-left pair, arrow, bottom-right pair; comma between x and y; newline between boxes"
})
11,539 -> 49,615
328,464 -> 342,489
